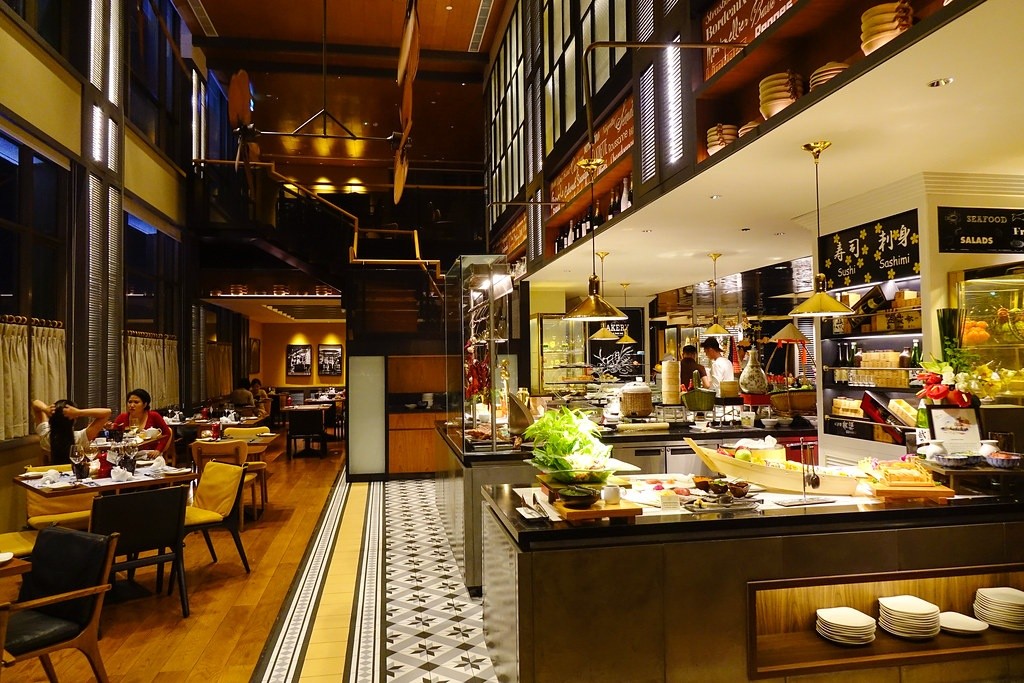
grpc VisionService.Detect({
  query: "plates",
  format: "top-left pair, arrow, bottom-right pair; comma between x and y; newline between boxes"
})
163,470 -> 190,474
0,552 -> 13,564
816,587 -> 1024,646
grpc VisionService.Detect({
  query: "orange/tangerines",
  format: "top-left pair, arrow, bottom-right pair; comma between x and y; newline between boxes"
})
962,320 -> 990,344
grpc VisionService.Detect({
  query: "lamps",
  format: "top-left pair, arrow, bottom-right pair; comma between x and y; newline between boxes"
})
616,282 -> 637,346
561,156 -> 630,323
704,252 -> 731,337
588,250 -> 619,343
788,139 -> 857,320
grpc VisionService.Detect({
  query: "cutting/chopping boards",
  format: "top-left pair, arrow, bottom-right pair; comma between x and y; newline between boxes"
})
553,498 -> 643,521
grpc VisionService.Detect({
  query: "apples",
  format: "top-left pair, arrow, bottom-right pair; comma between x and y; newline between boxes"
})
718,446 -> 751,462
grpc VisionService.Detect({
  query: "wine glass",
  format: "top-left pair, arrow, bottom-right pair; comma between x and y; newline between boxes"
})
69,440 -> 139,485
173,403 -> 235,423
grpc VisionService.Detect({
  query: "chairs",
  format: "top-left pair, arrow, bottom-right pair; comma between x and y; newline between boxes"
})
285,409 -> 329,460
186,440 -> 259,524
87,483 -> 194,641
165,459 -> 252,597
0,525 -> 121,683
221,426 -> 271,515
0,531 -> 38,558
155,424 -> 178,468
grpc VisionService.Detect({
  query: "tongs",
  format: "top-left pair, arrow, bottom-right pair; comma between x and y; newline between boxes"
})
521,492 -> 549,520
568,484 -> 597,497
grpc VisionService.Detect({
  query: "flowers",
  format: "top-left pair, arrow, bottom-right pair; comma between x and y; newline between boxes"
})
906,330 -> 1000,412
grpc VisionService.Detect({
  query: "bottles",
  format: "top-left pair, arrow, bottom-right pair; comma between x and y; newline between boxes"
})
910,339 -> 920,368
835,342 -> 862,367
898,346 -> 911,368
553,172 -> 633,254
925,440 -> 947,461
979,439 -> 1001,462
739,349 -> 767,394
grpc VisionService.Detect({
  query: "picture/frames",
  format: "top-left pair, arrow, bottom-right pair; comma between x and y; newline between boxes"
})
285,344 -> 312,376
317,343 -> 342,376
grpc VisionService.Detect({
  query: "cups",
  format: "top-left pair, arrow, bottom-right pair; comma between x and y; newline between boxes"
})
95,418 -> 162,446
739,412 -> 755,428
604,485 -> 627,506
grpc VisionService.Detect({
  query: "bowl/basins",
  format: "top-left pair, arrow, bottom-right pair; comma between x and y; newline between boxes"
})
934,452 -> 1024,470
706,3 -> 912,165
761,418 -> 778,427
777,418 -> 793,427
405,392 -> 433,409
557,489 -> 601,511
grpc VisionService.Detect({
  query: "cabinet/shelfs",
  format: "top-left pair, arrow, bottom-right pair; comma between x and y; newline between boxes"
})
819,276 -> 928,451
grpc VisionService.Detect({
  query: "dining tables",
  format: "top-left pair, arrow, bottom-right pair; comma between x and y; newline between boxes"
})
0,391 -> 346,642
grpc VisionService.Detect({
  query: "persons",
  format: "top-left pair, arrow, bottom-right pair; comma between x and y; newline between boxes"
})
33,398 -> 111,464
679,345 -> 709,392
229,378 -> 268,417
104,388 -> 171,465
701,337 -> 734,397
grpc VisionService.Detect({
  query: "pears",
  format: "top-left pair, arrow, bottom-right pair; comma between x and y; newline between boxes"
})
1001,308 -> 1024,343
